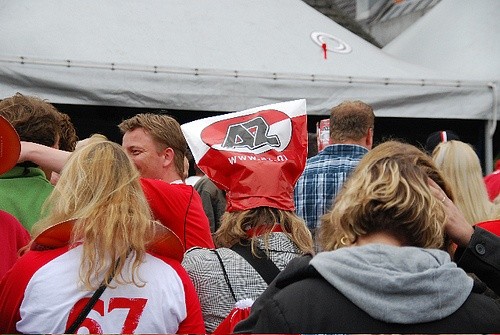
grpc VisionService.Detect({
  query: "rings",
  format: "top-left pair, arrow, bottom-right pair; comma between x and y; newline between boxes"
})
441,196 -> 445,202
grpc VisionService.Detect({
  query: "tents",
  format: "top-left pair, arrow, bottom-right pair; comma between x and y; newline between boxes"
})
1,0 -> 500,175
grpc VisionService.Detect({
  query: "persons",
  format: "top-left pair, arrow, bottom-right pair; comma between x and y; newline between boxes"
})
1,94 -> 499,335
